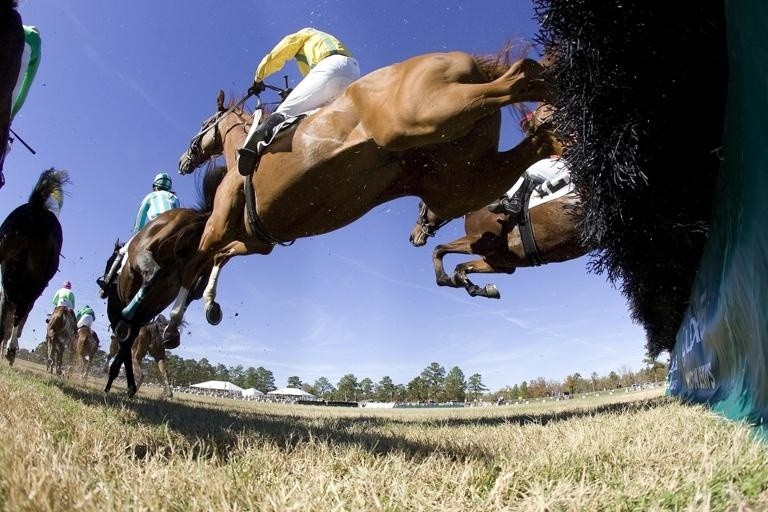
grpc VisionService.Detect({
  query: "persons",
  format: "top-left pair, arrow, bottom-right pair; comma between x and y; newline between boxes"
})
487,110 -> 575,223
132,172 -> 180,235
76,305 -> 95,333
46,172 -> 63,218
238,27 -> 360,176
46,280 -> 76,324
8,25 -> 41,124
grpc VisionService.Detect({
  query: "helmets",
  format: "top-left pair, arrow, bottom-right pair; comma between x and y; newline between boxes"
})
152,172 -> 172,190
519,110 -> 536,137
64,281 -> 71,289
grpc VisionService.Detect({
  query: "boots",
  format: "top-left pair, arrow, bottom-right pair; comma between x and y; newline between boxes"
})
96,254 -> 124,291
487,178 -> 537,217
238,113 -> 286,176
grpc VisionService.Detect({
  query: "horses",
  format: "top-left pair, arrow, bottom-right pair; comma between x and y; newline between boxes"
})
0,0 -> 611,401
